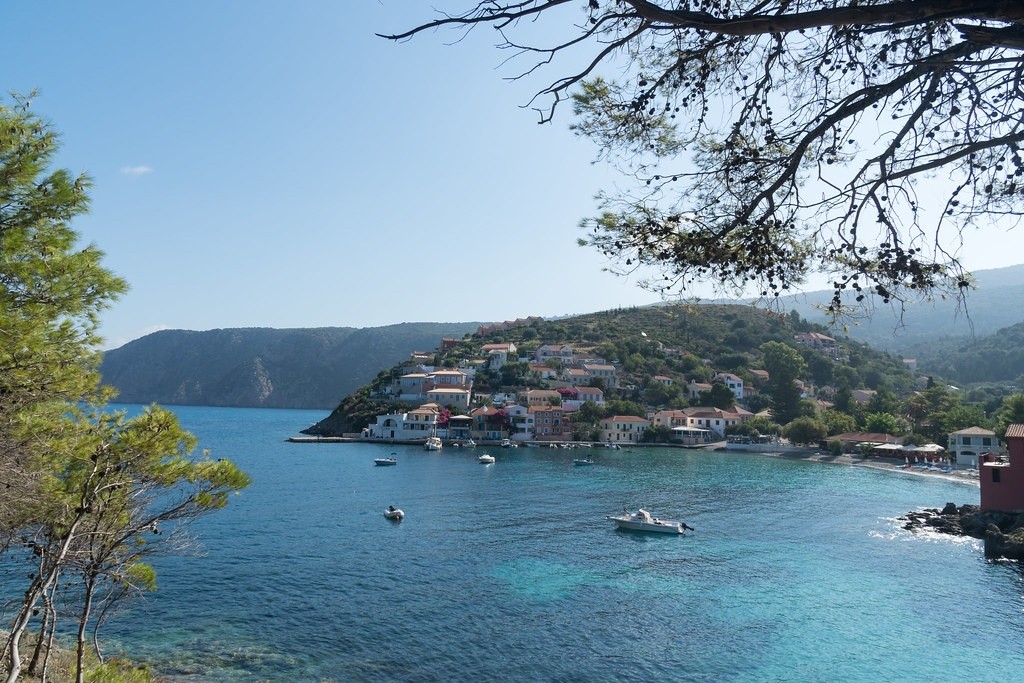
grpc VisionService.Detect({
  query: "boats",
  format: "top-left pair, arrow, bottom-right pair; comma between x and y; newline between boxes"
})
527,443 -> 540,448
424,435 -> 442,451
605,444 -> 621,450
479,454 -> 495,463
605,507 -> 694,534
573,454 -> 594,466
501,438 -> 518,449
549,443 -> 592,449
383,505 -> 404,520
453,443 -> 458,447
462,438 -> 476,448
374,452 -> 397,466
